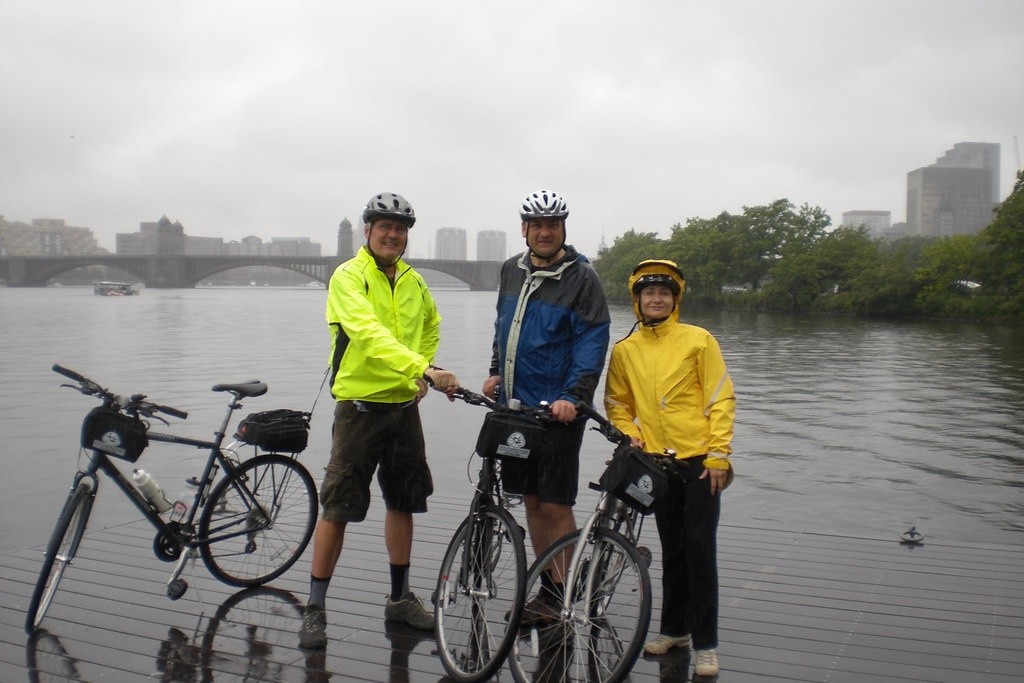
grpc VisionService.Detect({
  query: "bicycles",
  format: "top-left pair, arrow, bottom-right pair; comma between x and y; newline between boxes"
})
508,400 -> 691,683
24,364 -> 319,633
27,586 -> 326,683
430,383 -> 574,683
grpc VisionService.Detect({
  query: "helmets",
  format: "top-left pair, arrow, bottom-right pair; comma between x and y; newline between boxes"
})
632,274 -> 681,295
519,188 -> 569,220
362,192 -> 416,229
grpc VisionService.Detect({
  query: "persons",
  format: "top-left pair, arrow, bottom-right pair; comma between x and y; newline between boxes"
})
295,191 -> 457,640
480,189 -> 611,626
604,259 -> 737,676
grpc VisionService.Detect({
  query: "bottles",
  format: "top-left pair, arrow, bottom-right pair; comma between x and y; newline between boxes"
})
133,469 -> 169,513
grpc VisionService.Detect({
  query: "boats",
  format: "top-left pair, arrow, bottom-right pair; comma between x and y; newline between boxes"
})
95,282 -> 138,297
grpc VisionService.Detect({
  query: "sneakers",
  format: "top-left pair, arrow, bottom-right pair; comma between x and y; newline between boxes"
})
382,592 -> 435,629
643,635 -> 691,654
539,620 -> 574,648
298,603 -> 327,648
694,649 -> 719,675
505,597 -> 562,625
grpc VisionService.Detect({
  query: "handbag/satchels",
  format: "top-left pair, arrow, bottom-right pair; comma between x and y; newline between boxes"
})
474,411 -> 553,463
238,409 -> 312,454
599,444 -> 671,516
82,407 -> 149,463
